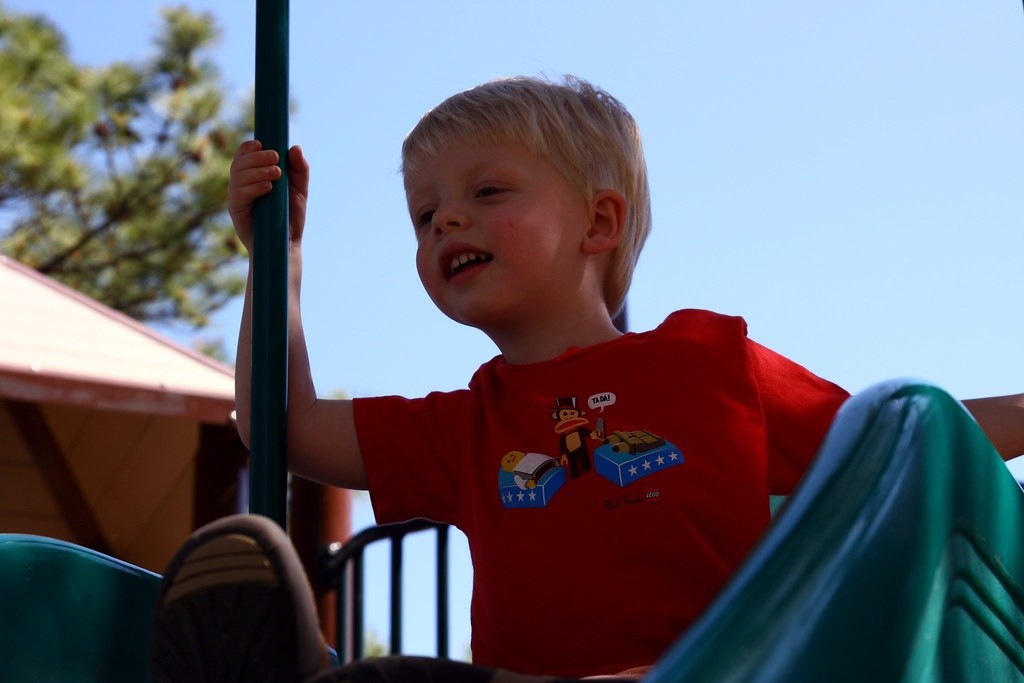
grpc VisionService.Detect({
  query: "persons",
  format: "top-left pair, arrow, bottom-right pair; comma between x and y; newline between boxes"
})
228,74 -> 1024,683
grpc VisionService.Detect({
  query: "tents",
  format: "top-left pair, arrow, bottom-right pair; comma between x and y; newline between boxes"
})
0,255 -> 352,654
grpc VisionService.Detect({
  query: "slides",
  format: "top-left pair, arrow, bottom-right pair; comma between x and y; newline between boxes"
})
0,371 -> 1023,682
